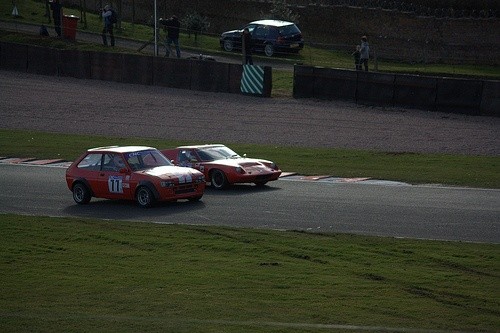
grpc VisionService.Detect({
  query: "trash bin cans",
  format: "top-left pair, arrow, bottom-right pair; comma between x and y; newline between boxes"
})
62,15 -> 80,41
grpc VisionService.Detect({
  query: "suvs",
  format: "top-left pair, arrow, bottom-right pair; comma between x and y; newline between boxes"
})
219,18 -> 305,57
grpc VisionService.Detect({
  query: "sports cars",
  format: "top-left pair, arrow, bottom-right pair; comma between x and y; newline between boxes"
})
65,146 -> 206,208
144,143 -> 282,190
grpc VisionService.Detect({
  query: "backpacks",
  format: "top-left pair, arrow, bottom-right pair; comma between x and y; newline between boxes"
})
107,9 -> 118,24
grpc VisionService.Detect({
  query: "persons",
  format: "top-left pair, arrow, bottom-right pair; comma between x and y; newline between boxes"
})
101,6 -> 115,46
242,28 -> 253,64
351,36 -> 368,70
158,14 -> 181,58
51,0 -> 61,38
112,157 -> 132,173
